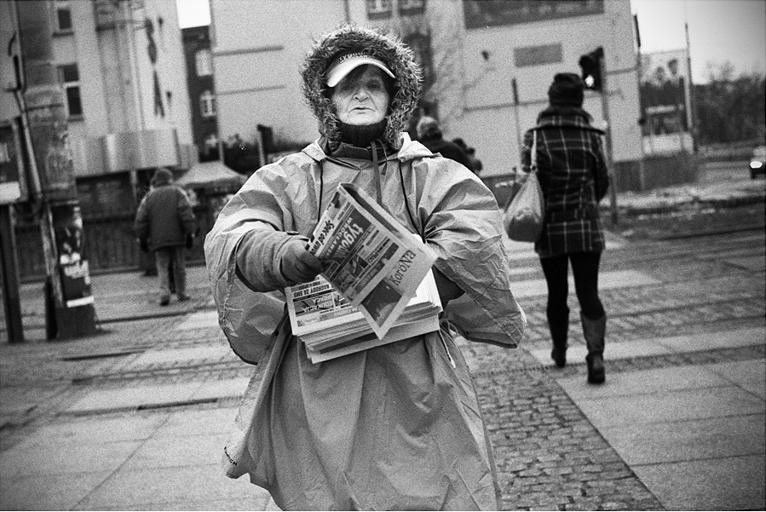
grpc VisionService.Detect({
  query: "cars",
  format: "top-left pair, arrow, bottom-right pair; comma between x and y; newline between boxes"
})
748,156 -> 766,178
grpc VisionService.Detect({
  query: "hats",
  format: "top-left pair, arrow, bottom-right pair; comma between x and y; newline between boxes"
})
325,55 -> 396,88
548,73 -> 585,107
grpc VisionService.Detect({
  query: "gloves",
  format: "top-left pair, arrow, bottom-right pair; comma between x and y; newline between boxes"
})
281,240 -> 322,283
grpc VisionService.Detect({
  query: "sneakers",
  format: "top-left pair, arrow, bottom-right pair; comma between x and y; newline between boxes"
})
159,294 -> 190,305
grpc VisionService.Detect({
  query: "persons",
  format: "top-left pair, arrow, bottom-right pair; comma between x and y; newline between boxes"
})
129,164 -> 198,307
413,116 -> 473,178
202,25 -> 529,509
517,73 -> 610,385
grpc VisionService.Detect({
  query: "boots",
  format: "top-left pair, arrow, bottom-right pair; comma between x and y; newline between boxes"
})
546,305 -> 570,368
580,311 -> 606,383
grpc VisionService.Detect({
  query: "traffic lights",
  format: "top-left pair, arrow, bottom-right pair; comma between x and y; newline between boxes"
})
578,54 -> 600,91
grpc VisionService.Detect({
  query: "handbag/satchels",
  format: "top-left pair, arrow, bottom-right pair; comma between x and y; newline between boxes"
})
504,171 -> 545,242
